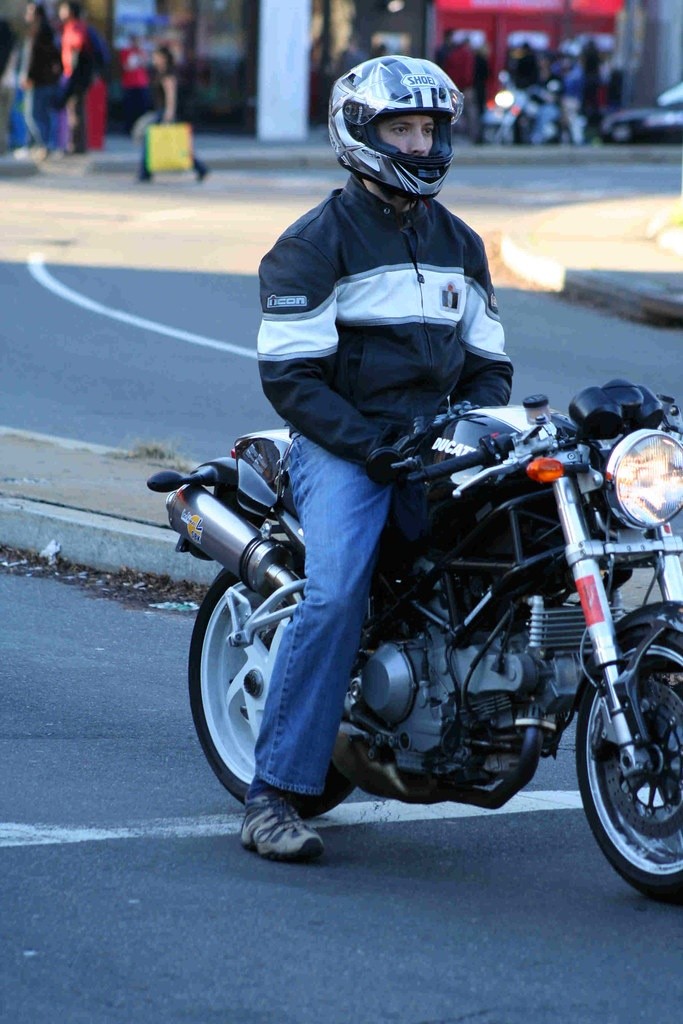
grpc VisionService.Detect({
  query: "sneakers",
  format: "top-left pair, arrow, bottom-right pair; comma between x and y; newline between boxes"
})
239,791 -> 323,859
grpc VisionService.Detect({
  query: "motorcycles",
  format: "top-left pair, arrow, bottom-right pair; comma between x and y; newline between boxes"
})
147,379 -> 683,904
483,71 -> 588,147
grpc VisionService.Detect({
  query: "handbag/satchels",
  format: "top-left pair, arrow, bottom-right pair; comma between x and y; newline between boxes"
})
145,120 -> 194,172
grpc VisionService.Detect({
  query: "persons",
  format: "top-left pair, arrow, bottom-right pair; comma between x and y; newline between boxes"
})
120,32 -> 181,126
0,0 -> 95,161
433,27 -> 605,143
136,48 -> 210,183
340,36 -> 388,75
238,58 -> 516,862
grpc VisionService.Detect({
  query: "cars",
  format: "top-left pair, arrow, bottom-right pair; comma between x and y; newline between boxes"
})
599,84 -> 682,145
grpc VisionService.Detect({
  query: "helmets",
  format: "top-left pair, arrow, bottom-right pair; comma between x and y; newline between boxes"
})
327,54 -> 464,199
